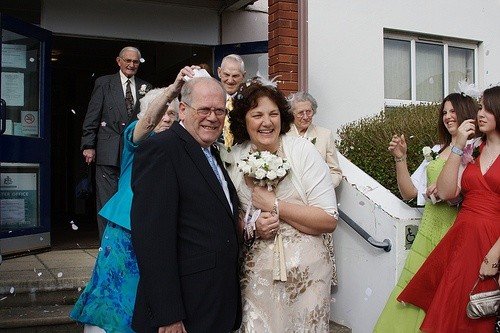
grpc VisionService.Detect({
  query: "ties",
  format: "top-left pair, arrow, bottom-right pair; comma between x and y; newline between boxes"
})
126,80 -> 134,119
203,148 -> 222,185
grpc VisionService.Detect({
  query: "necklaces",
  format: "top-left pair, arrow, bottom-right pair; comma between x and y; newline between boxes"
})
450,142 -> 454,147
485,142 -> 498,167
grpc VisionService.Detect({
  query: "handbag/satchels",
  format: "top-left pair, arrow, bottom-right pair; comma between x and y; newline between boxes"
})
466,276 -> 500,320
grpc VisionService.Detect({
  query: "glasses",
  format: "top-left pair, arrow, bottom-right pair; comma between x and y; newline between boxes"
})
296,111 -> 314,118
183,101 -> 226,117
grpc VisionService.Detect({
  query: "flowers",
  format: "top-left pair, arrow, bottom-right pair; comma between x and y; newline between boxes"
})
422,145 -> 439,162
461,142 -> 481,165
237,149 -> 289,231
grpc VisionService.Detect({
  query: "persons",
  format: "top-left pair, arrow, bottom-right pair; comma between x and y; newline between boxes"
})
397,86 -> 500,333
371,81 -> 482,333
68,64 -> 199,333
130,76 -> 244,333
228,82 -> 338,333
284,91 -> 342,188
215,55 -> 246,152
81,46 -> 152,247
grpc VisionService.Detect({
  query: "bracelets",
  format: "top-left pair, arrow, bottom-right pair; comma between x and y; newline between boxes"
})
273,198 -> 281,214
452,145 -> 464,156
164,84 -> 175,101
483,256 -> 500,268
394,154 -> 407,162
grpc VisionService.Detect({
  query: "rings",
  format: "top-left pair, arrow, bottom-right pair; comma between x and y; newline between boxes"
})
465,127 -> 468,132
272,229 -> 276,236
179,71 -> 185,75
388,147 -> 391,151
